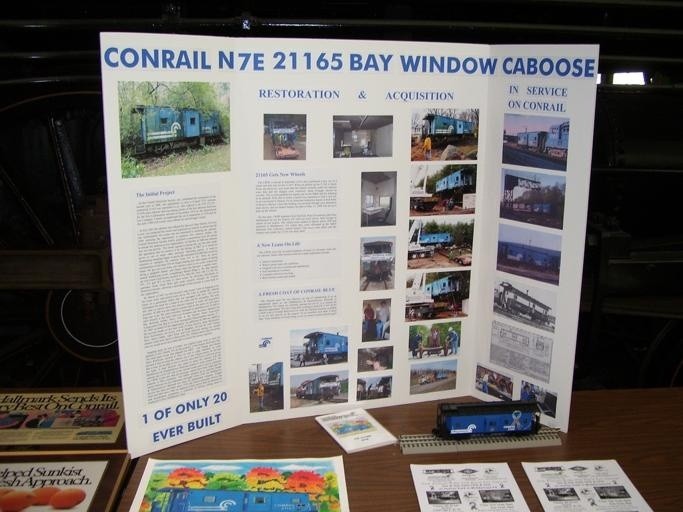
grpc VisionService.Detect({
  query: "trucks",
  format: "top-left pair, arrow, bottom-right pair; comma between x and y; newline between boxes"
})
267,362 -> 283,386
517,121 -> 568,160
131,105 -> 222,155
406,168 -> 551,325
296,332 -> 347,400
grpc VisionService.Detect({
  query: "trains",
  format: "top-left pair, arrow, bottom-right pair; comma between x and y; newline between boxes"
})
362,240 -> 395,280
423,114 -> 472,149
151,488 -> 318,512
337,425 -> 368,433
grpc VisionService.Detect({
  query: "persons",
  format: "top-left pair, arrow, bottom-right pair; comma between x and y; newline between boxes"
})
520,384 -> 536,401
421,134 -> 432,161
481,372 -> 489,394
363,302 -> 374,338
410,326 -> 458,359
365,352 -> 393,371
356,380 -> 389,399
298,353 -> 305,367
373,300 -> 389,339
253,381 -> 265,410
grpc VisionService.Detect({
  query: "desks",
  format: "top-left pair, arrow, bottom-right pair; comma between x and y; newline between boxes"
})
0,387 -> 133,512
116,387 -> 683,512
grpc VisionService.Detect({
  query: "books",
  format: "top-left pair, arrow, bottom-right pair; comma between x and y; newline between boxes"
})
313,406 -> 398,455
